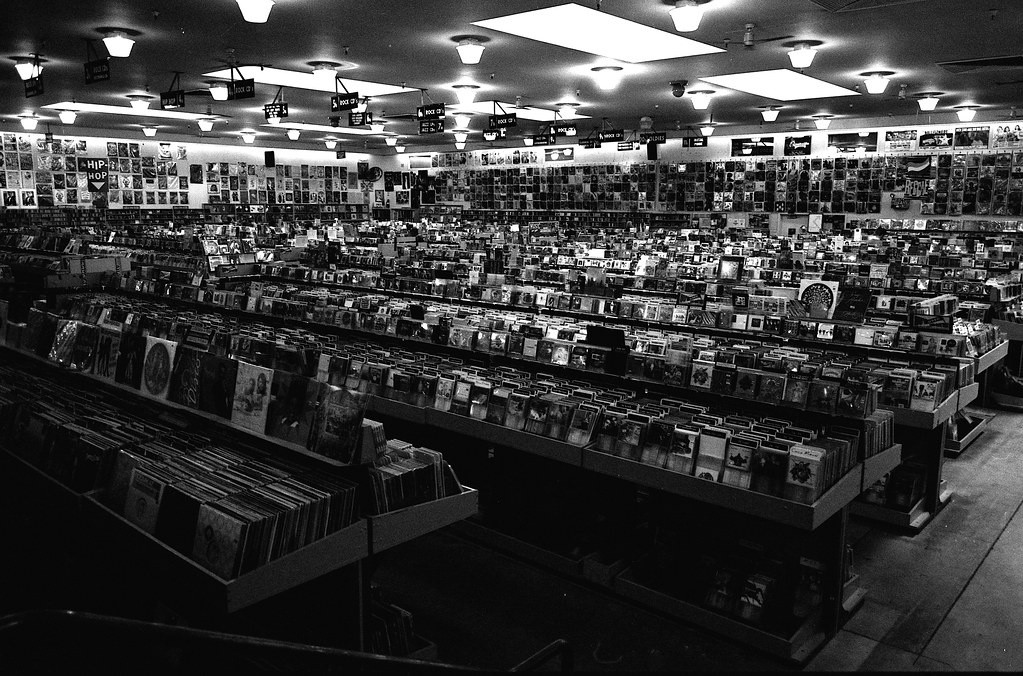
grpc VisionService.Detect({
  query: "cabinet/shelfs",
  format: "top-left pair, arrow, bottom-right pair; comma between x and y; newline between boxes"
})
1,202 -> 1023,676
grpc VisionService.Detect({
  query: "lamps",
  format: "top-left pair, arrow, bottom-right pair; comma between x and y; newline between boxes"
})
8,0 -> 979,160
